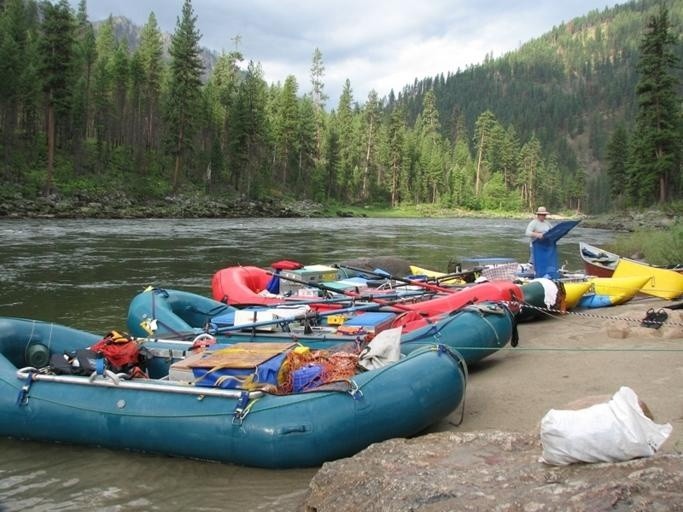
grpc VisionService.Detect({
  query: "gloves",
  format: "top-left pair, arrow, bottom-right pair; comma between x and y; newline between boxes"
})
446,256 -> 598,283
1,317 -> 469,468
409,264 -> 593,311
578,242 -> 683,299
331,257 -> 565,325
457,257 -> 656,308
337,257 -> 566,320
578,276 -> 654,309
1,317 -> 468,469
128,285 -> 518,367
579,240 -> 683,278
407,264 -> 593,311
212,260 -> 523,322
126,286 -> 520,367
210,264 -> 525,334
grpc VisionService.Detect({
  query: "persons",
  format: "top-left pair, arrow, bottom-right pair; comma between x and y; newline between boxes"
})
524,207 -> 553,275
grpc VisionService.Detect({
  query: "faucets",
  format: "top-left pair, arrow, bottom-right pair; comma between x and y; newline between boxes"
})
640,307 -> 669,330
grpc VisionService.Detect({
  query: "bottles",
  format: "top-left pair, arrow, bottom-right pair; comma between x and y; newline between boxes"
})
535,206 -> 549,215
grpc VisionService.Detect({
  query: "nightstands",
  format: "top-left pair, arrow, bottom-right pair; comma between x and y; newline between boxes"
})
147,293 -> 435,341
